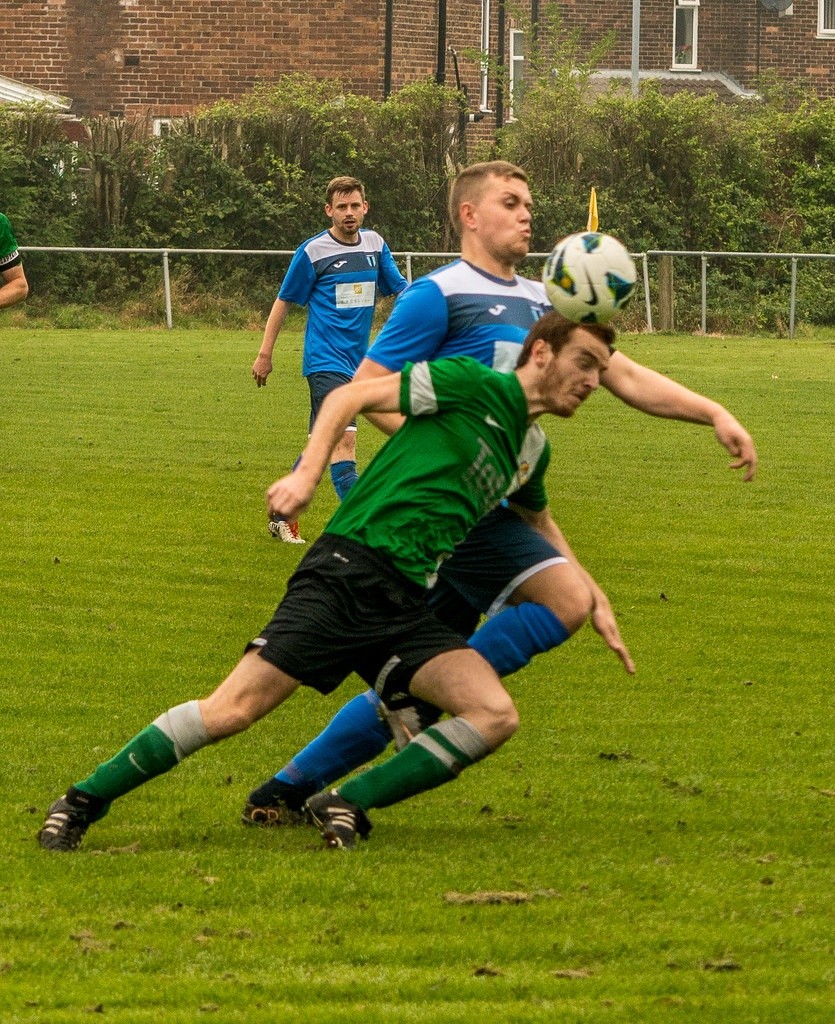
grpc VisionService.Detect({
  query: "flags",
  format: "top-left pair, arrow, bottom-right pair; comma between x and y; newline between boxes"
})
587,189 -> 599,232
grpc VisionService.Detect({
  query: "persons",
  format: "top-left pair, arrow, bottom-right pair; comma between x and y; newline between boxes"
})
244,161 -> 757,821
35,311 -> 616,853
253,176 -> 410,504
0,213 -> 29,309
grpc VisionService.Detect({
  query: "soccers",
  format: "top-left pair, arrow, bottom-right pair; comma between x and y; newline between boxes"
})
541,233 -> 635,323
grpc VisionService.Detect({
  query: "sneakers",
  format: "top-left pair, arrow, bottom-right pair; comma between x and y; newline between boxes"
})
240,785 -> 314,829
268,519 -> 306,544
303,788 -> 372,852
37,789 -> 109,852
378,692 -> 439,751
